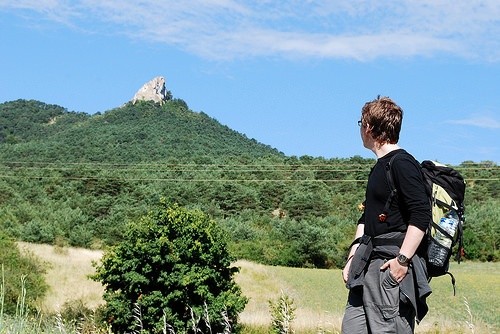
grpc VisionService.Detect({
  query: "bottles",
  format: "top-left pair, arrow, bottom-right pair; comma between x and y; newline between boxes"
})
427,206 -> 459,266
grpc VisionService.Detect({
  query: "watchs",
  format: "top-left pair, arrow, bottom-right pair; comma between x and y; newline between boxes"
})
397,253 -> 410,264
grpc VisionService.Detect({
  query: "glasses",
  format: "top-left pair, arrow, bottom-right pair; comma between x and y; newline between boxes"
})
358,121 -> 367,127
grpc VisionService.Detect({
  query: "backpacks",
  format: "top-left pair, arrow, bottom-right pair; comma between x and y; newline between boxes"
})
385,151 -> 466,276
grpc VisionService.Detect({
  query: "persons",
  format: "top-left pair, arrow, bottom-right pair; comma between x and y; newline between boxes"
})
340,94 -> 432,334
